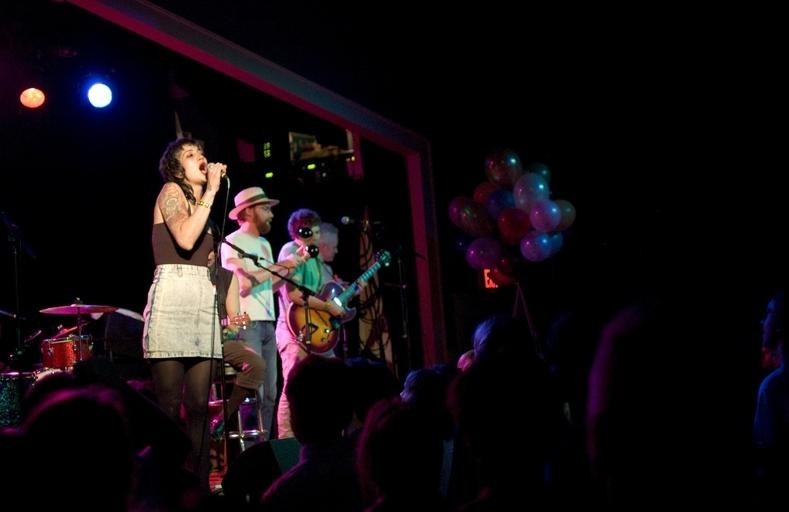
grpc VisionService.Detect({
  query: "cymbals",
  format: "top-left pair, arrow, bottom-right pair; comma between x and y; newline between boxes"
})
52,322 -> 87,336
39,304 -> 118,315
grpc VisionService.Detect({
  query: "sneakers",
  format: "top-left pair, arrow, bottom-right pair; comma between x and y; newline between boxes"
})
208,416 -> 225,449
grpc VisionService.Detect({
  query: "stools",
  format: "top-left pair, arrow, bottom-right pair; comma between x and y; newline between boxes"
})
211,362 -> 267,451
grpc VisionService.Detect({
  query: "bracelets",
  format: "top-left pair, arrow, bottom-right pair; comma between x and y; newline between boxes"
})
196,201 -> 211,208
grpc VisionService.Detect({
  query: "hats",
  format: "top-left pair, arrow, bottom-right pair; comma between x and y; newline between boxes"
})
228,187 -> 280,221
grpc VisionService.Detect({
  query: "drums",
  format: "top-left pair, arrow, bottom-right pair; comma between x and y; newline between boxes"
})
29,369 -> 126,432
41,334 -> 92,369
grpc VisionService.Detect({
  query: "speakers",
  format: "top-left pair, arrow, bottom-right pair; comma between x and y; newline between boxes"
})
221,437 -> 301,499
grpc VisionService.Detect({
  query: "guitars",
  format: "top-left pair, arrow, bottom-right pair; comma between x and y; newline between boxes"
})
285,249 -> 393,352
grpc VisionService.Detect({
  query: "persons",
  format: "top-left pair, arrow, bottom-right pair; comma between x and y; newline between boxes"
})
275,208 -> 367,441
317,222 -> 349,287
143,136 -> 221,452
221,187 -> 310,441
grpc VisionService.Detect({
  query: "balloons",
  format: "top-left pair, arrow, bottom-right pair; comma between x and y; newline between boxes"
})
448,146 -> 577,276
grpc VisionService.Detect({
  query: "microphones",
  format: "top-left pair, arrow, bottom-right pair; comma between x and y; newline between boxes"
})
206,163 -> 228,179
341,217 -> 364,226
238,253 -> 265,260
